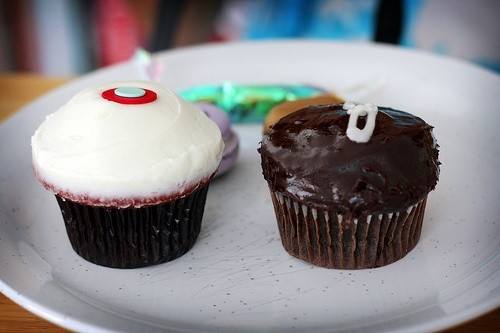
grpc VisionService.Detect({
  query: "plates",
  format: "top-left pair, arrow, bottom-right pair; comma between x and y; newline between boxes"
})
0,35 -> 498,333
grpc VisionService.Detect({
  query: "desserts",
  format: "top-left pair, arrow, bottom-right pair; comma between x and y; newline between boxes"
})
193,100 -> 239,183
30,80 -> 225,269
263,94 -> 347,137
257,102 -> 443,270
